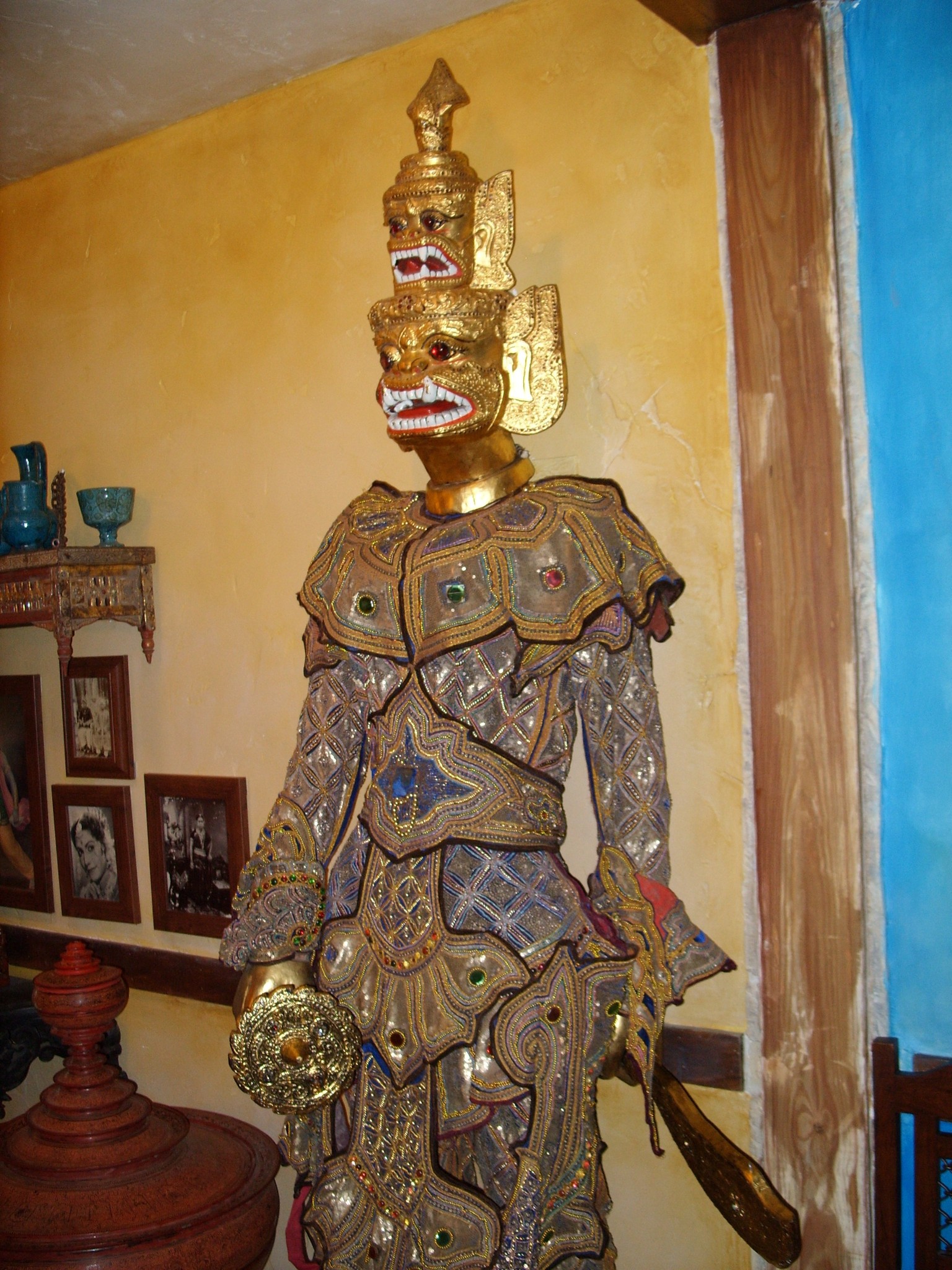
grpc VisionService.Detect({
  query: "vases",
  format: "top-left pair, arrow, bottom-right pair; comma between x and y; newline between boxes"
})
2,481 -> 52,551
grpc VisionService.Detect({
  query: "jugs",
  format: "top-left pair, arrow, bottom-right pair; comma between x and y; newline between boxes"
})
0,480 -> 51,555
9,442 -> 57,551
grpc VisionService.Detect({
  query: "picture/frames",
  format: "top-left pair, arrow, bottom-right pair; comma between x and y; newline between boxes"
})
60,654 -> 136,779
143,772 -> 250,939
51,784 -> 142,925
0,674 -> 55,914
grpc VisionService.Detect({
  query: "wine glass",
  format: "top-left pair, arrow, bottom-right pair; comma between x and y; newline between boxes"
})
75,486 -> 135,548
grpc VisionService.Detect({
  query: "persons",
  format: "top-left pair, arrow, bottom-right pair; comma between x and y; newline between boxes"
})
217,57 -> 738,1270
70,812 -> 117,899
188,813 -> 212,914
77,697 -> 111,758
0,748 -> 35,888
